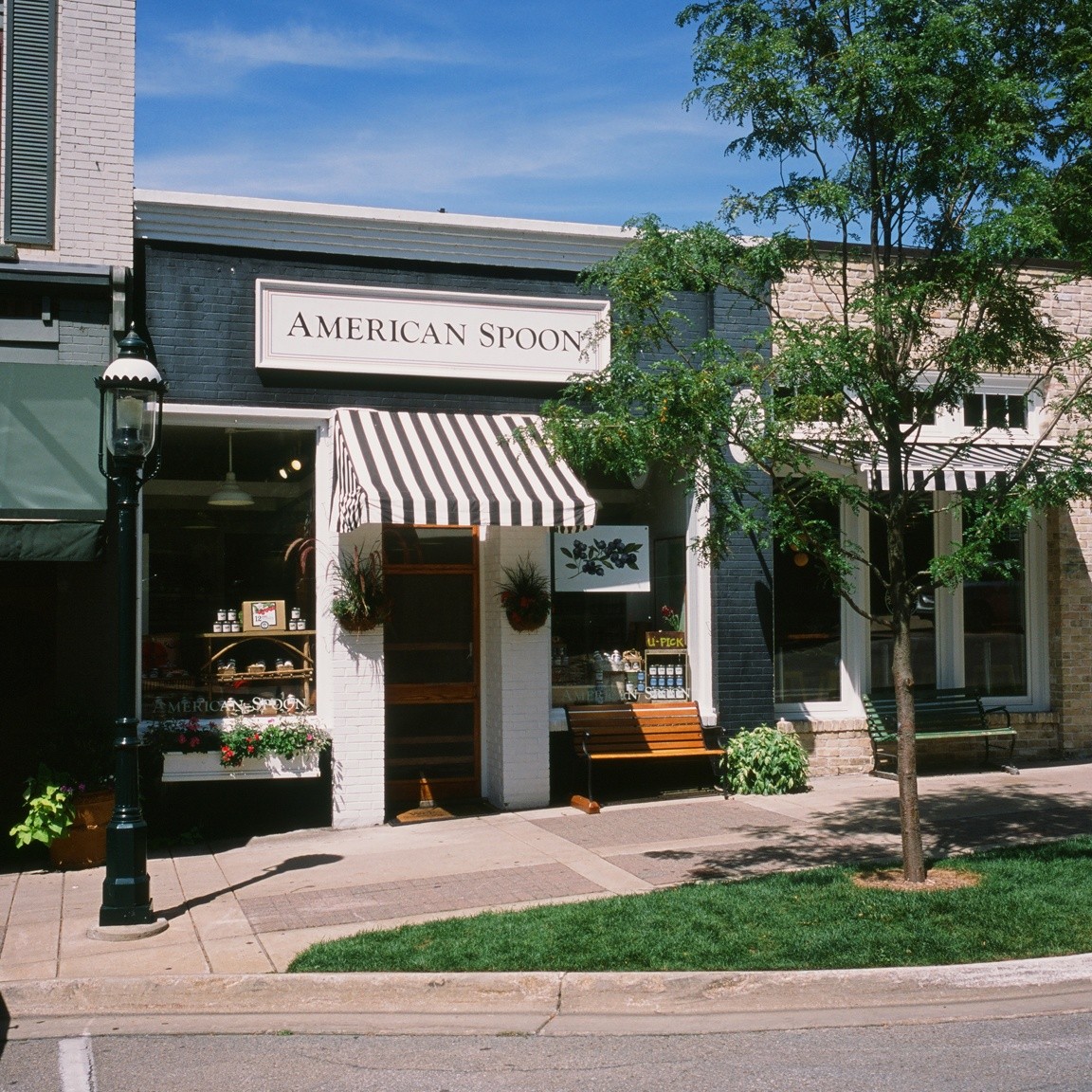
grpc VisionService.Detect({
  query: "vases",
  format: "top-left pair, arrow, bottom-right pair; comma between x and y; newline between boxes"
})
505,605 -> 549,632
150,745 -> 323,782
46,786 -> 116,871
646,630 -> 685,649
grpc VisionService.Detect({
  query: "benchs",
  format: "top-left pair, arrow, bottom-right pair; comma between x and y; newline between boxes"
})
563,699 -> 728,814
860,685 -> 1021,781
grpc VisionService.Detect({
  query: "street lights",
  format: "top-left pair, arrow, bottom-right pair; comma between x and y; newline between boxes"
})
94,321 -> 169,928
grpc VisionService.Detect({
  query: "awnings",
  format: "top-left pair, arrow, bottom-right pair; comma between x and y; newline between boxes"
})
790,442 -> 1092,491
330,410 -> 596,533
0,361 -> 108,561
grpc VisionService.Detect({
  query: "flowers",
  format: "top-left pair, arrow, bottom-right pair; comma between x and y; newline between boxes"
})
491,549 -> 558,635
139,713 -> 331,769
6,762 -> 149,851
660,605 -> 682,632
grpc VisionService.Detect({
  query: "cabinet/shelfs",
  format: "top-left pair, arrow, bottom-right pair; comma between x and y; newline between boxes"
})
194,628 -> 317,716
642,648 -> 688,702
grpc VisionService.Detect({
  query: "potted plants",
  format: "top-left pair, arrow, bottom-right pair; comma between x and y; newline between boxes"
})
282,533 -> 392,645
